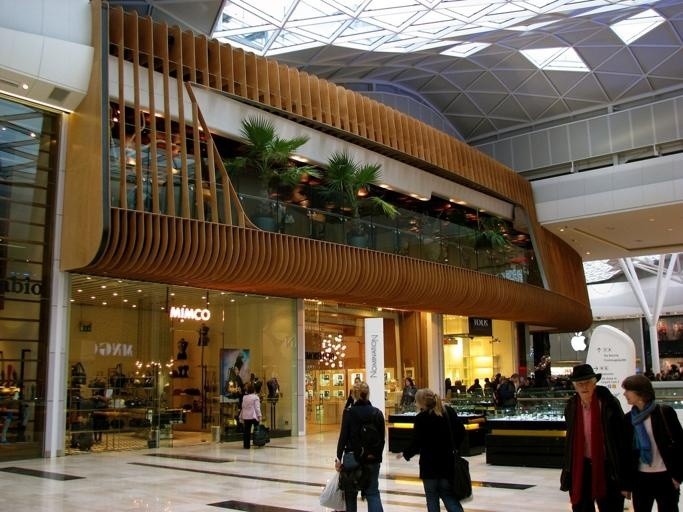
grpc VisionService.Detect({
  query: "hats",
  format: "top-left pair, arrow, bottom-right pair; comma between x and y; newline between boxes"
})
567,364 -> 602,383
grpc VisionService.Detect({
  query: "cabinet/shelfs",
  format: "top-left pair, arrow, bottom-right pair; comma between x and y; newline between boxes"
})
306,365 -> 683,470
0,353 -> 281,452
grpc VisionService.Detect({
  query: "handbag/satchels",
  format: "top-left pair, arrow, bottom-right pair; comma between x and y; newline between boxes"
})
110,374 -> 126,387
338,452 -> 364,491
253,427 -> 270,446
447,457 -> 471,501
71,373 -> 87,385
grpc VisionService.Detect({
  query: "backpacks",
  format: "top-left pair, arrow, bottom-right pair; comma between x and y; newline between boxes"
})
350,408 -> 385,463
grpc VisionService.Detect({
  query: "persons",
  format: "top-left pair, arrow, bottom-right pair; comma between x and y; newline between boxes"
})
90,378 -> 110,444
345,377 -> 361,408
403,389 -> 469,512
644,365 -> 683,381
399,378 -> 417,414
335,382 -> 385,512
239,386 -> 262,449
560,364 -> 625,512
446,374 -> 536,410
620,375 -> 683,512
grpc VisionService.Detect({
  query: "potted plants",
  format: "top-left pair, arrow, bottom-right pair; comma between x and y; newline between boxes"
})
204,111 -> 318,238
297,144 -> 404,252
